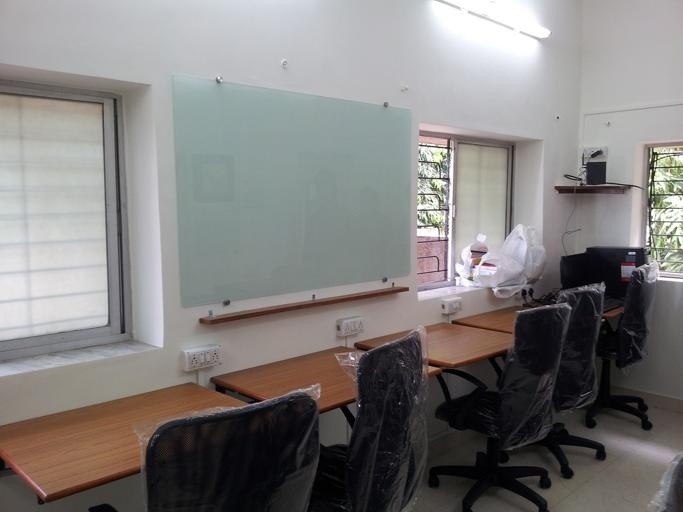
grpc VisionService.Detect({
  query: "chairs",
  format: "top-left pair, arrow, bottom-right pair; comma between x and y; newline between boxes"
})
583,259 -> 653,432
135,390 -> 321,512
430,303 -> 573,512
503,282 -> 607,479
322,328 -> 433,510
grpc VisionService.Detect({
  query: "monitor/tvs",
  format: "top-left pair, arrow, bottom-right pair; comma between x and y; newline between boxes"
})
560,251 -> 616,294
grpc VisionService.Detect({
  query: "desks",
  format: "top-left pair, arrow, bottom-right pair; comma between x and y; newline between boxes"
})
208,343 -> 440,434
352,320 -> 515,401
453,305 -> 537,335
0,381 -> 248,506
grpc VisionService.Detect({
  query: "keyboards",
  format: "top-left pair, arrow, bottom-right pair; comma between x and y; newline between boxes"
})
603,297 -> 624,313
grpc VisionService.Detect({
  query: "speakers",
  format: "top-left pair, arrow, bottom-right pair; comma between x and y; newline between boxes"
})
586,162 -> 606,185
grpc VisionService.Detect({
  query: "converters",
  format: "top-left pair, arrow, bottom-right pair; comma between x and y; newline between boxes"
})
590,150 -> 602,158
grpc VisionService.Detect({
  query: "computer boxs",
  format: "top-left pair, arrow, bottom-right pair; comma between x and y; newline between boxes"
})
585,246 -> 645,287
